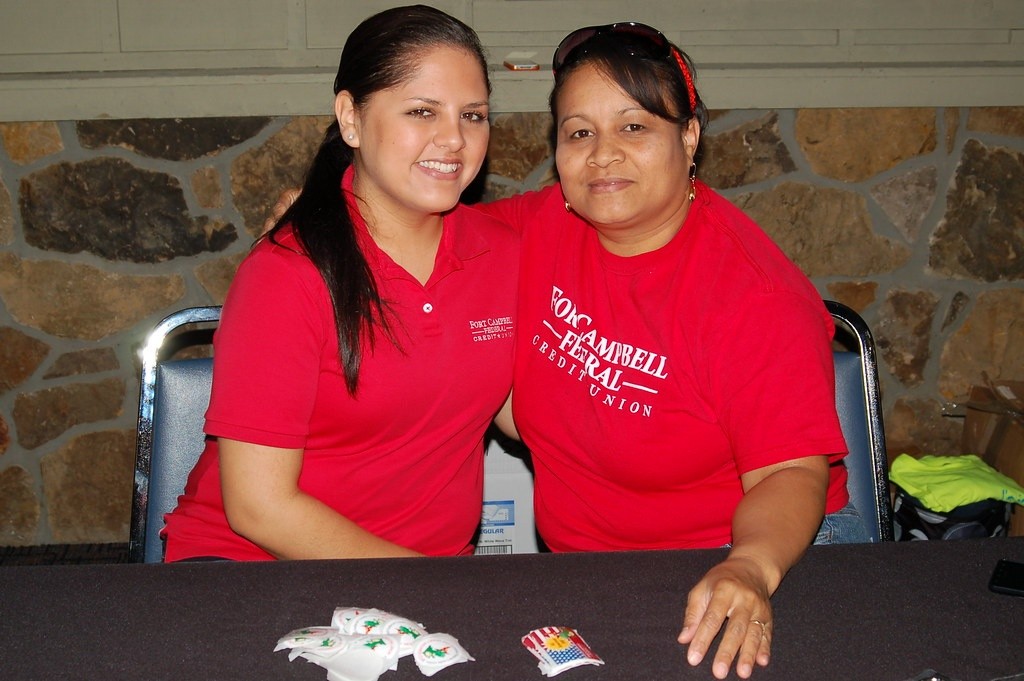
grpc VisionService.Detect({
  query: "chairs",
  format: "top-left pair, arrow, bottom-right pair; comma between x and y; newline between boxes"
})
123,303 -> 230,562
808,299 -> 896,543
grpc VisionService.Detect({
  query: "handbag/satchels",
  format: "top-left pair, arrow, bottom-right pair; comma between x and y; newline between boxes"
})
892,486 -> 1015,542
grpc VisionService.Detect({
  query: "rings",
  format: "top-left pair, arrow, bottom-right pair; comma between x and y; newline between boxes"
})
749,620 -> 765,634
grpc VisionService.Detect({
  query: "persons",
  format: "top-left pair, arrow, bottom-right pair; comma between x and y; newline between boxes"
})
255,21 -> 850,679
159,4 -> 519,563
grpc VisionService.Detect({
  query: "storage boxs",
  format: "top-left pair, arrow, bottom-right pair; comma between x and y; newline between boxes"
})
944,369 -> 1024,536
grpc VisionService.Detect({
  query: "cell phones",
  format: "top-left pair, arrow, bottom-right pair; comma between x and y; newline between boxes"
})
502,59 -> 540,71
989,559 -> 1024,596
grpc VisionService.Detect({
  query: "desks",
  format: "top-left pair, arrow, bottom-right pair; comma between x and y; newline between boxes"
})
0,530 -> 1024,681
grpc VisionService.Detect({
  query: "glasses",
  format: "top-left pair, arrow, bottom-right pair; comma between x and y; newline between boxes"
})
553,22 -> 697,116
